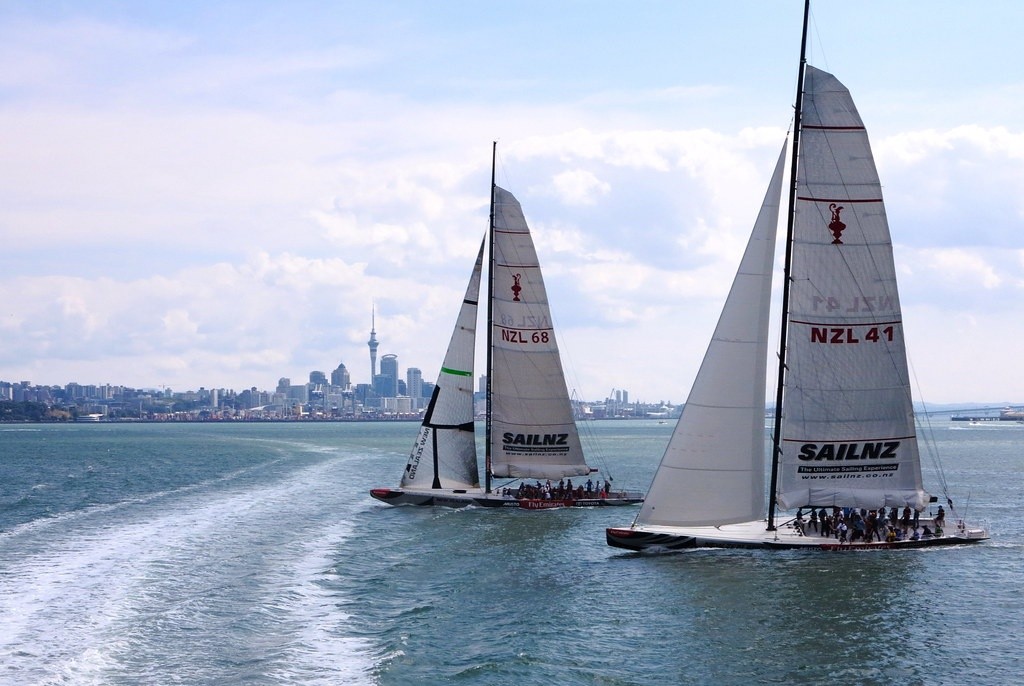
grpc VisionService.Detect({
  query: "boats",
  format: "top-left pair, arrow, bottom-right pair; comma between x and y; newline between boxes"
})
658,421 -> 668,424
970,421 -> 981,426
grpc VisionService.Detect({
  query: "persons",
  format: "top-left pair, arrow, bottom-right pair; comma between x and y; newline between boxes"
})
797,505 -> 945,543
506,479 -> 611,501
958,520 -> 965,533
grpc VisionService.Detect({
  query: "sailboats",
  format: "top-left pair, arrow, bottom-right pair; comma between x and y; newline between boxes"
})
370,140 -> 646,510
608,0 -> 987,551
